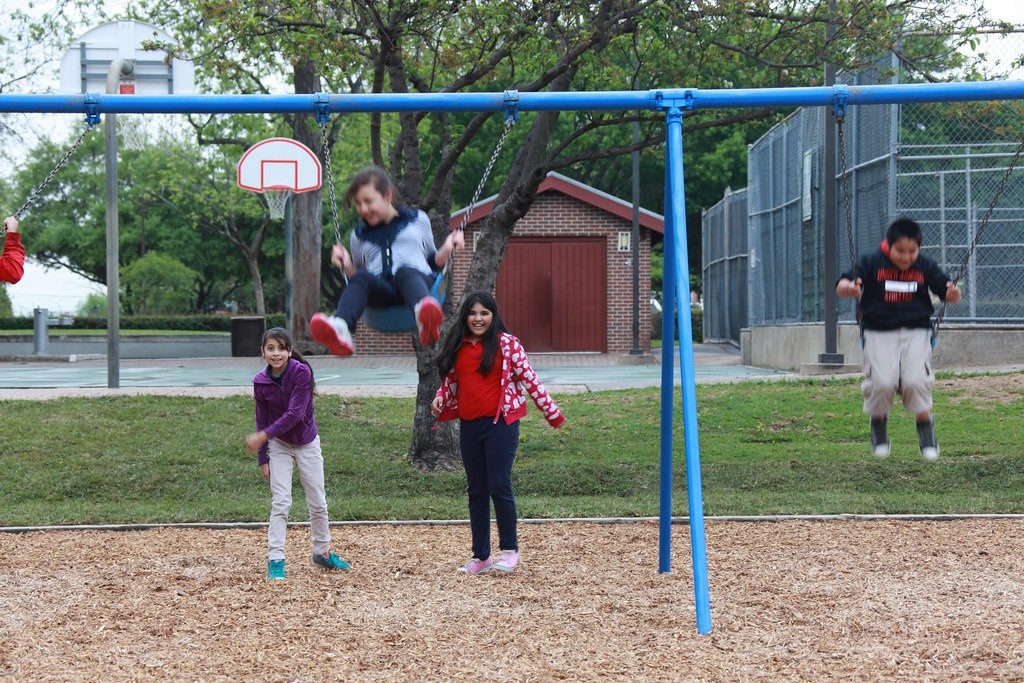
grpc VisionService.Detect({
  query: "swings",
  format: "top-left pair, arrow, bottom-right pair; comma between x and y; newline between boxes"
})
317,109 -> 519,332
0,110 -> 100,239
833,105 -> 1024,349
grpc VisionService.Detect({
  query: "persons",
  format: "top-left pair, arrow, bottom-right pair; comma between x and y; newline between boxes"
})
431,292 -> 568,574
245,327 -> 351,579
834,218 -> 961,460
309,164 -> 465,356
0,215 -> 25,284
